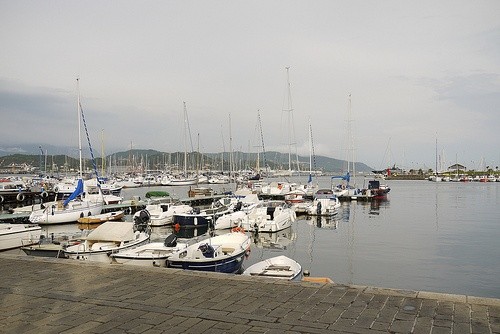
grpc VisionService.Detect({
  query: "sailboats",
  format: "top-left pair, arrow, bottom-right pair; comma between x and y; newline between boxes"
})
0,67 -> 391,233
427,138 -> 500,182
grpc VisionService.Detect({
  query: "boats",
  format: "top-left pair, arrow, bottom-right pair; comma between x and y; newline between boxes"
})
19,228 -> 97,257
110,234 -> 189,267
165,226 -> 252,274
0,223 -> 42,250
241,255 -> 303,281
57,220 -> 152,262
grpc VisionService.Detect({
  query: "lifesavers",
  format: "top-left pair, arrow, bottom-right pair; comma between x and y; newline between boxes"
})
16,193 -> 24,201
53,186 -> 59,193
0,195 -> 4,203
230,227 -> 246,234
41,192 -> 48,198
